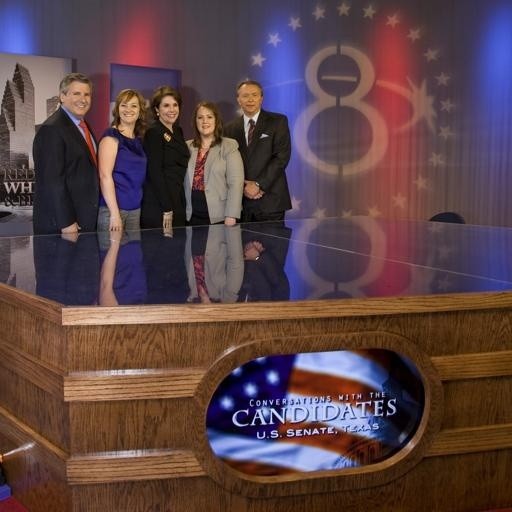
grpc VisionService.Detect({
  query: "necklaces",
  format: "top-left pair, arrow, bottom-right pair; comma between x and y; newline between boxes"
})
201,145 -> 211,149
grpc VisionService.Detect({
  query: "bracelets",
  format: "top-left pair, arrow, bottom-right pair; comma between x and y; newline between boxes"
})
255,181 -> 261,187
109,239 -> 121,244
110,216 -> 121,219
255,257 -> 260,262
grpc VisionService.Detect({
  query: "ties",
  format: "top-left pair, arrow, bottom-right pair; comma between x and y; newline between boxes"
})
247,119 -> 256,145
79,119 -> 97,169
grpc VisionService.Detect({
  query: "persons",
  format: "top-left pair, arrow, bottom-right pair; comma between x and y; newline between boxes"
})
97,231 -> 148,306
141,227 -> 191,305
182,100 -> 246,224
141,84 -> 191,229
222,79 -> 295,222
32,234 -> 100,306
97,88 -> 150,231
29,69 -> 98,234
237,221 -> 292,302
183,224 -> 245,305
